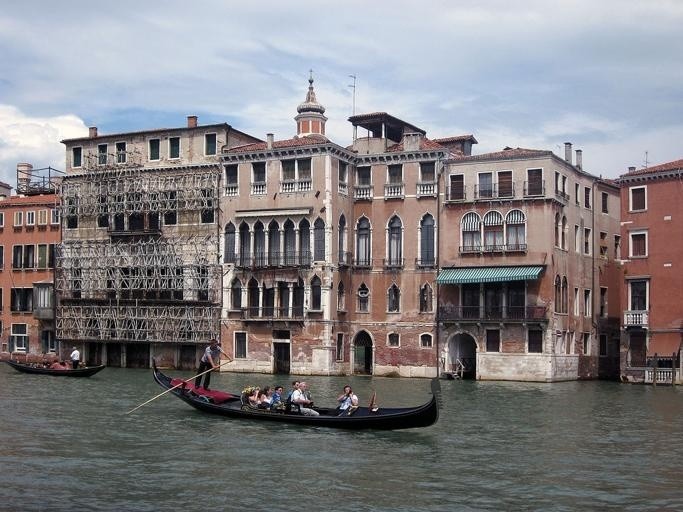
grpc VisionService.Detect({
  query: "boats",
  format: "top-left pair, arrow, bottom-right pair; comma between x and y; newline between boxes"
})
151,357 -> 437,430
7,359 -> 105,378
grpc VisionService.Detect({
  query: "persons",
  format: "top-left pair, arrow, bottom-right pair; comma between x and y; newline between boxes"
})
337,386 -> 359,417
43,346 -> 81,370
194,338 -> 232,391
247,380 -> 320,416
